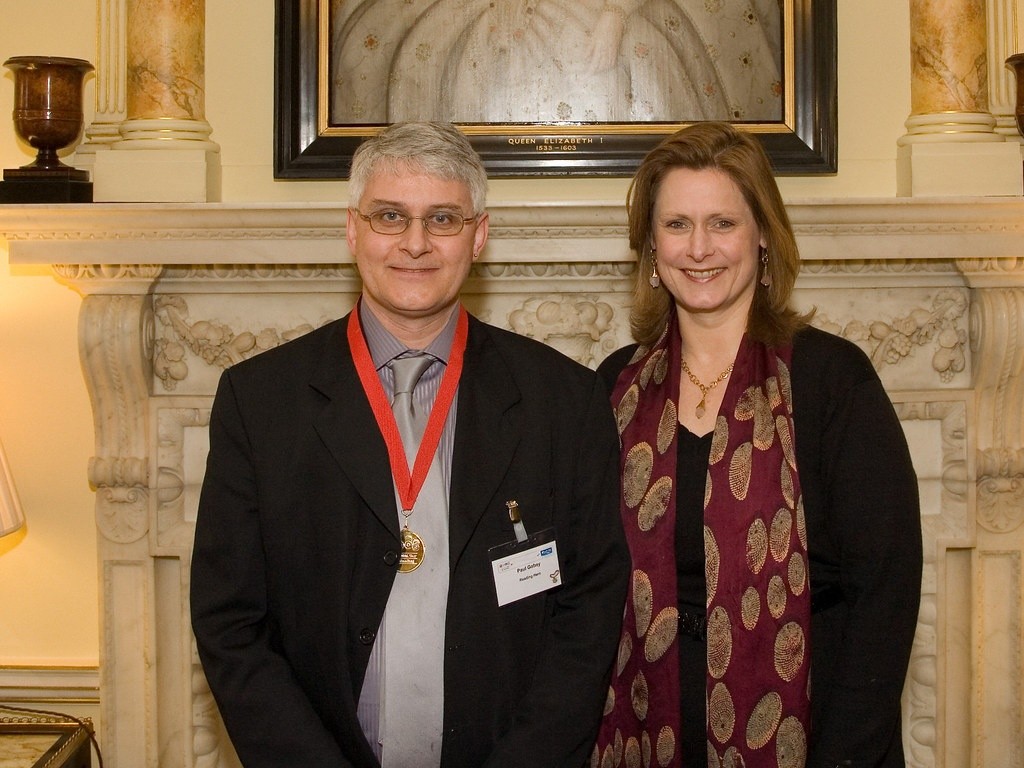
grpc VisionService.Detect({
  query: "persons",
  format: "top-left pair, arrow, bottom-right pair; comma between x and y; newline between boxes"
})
188,126 -> 627,768
598,126 -> 923,768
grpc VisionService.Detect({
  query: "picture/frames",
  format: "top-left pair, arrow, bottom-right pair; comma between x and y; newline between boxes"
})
272,0 -> 837,179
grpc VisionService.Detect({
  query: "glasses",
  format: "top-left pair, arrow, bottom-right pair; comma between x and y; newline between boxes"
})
354,207 -> 479,237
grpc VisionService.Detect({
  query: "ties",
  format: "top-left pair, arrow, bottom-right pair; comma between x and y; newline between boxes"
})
378,355 -> 448,768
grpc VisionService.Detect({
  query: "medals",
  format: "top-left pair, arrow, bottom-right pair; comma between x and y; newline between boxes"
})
396,525 -> 425,574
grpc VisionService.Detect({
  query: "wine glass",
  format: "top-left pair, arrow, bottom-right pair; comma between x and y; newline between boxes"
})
0,54 -> 96,178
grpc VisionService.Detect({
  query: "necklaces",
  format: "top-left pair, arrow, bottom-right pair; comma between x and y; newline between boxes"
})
681,354 -> 740,418
